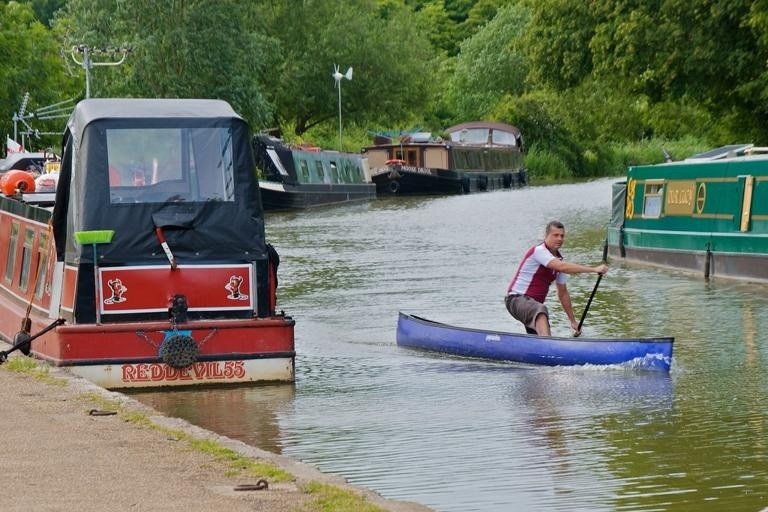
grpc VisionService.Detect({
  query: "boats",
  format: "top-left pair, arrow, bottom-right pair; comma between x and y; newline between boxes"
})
396,308 -> 675,372
361,120 -> 529,194
252,127 -> 377,208
608,144 -> 768,281
1,99 -> 297,389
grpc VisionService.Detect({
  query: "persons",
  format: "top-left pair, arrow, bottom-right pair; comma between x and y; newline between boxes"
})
504,221 -> 609,337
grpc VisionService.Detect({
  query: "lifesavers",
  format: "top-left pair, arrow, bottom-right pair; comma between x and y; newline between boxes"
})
385,160 -> 406,166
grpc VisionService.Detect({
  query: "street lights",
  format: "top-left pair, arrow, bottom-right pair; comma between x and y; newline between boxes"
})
60,44 -> 137,99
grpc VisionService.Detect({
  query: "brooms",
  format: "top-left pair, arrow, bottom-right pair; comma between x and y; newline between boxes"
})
75,230 -> 115,324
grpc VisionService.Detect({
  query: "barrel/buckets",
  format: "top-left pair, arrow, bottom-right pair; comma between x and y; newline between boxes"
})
37,179 -> 55,193
0,169 -> 36,196
